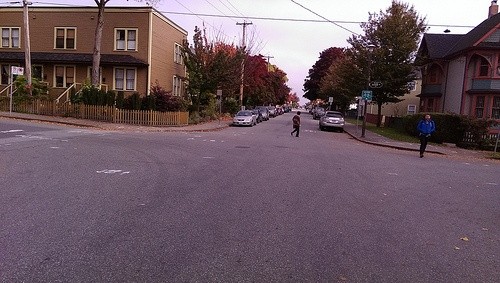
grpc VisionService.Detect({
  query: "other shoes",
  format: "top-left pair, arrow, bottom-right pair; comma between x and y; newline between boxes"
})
420,154 -> 424,158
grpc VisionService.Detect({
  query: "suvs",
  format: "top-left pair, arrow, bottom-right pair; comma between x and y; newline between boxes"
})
318,111 -> 345,133
268,106 -> 277,118
256,107 -> 270,121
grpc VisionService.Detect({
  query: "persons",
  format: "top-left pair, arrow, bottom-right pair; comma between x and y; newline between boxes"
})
291,111 -> 301,137
416,113 -> 435,158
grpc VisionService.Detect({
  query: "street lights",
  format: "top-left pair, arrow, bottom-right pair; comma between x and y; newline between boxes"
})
366,41 -> 376,87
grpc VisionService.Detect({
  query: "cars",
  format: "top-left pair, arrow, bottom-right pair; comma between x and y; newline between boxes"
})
304,104 -> 316,115
252,109 -> 263,123
275,105 -> 292,115
313,110 -> 326,120
232,111 -> 257,127
